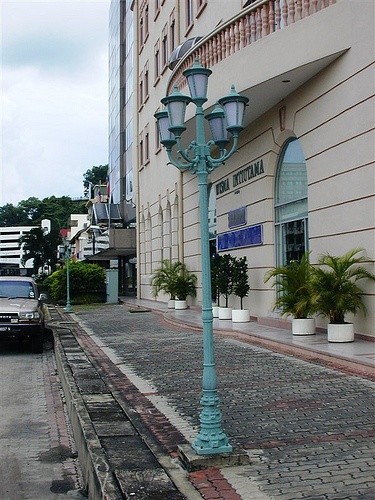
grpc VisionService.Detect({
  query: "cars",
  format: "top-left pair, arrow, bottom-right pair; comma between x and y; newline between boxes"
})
0,275 -> 49,354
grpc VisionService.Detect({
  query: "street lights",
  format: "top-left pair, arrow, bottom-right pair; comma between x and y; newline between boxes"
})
63,236 -> 74,312
153,55 -> 252,455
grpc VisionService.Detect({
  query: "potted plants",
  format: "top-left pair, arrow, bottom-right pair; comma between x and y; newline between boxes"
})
263,250 -> 320,336
149,255 -> 198,309
295,247 -> 375,343
210,250 -> 252,323
